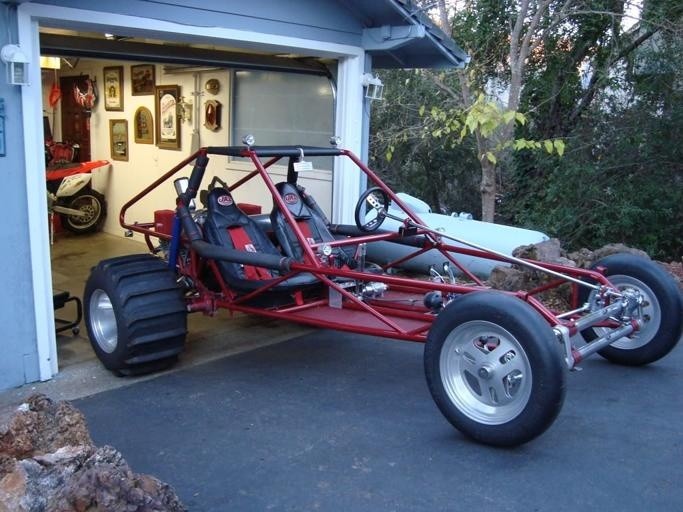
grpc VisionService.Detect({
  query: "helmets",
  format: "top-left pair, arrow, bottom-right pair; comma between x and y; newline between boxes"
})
73,79 -> 97,108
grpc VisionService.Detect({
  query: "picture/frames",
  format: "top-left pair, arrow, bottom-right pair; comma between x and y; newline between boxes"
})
101,63 -> 180,162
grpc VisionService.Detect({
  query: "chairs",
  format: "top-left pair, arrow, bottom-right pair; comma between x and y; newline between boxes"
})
199,185 -> 332,290
270,184 -> 389,281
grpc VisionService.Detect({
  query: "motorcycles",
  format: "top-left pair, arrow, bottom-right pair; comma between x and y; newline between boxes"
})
45,158 -> 109,245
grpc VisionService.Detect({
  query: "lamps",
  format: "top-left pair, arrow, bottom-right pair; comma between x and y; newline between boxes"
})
177,97 -> 192,123
1,43 -> 32,88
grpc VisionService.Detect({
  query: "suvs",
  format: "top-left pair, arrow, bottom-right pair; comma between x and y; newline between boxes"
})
83,135 -> 682,448
